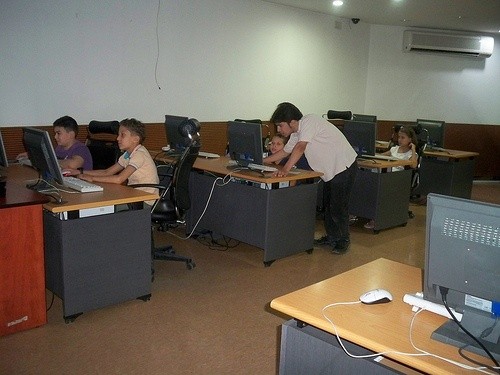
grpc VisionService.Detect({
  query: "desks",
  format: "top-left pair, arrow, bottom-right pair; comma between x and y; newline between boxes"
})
39,167 -> 160,324
149,150 -> 324,267
349,159 -> 417,234
0,162 -> 51,337
270,257 -> 500,375
376,141 -> 479,199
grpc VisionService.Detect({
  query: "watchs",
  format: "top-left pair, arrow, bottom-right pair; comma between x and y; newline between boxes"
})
77,167 -> 83,175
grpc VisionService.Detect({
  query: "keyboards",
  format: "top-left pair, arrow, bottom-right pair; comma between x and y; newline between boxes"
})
363,154 -> 399,160
229,160 -> 278,171
20,159 -> 104,193
162,147 -> 220,157
403,292 -> 462,323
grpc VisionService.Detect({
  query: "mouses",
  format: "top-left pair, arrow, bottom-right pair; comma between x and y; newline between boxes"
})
359,289 -> 392,305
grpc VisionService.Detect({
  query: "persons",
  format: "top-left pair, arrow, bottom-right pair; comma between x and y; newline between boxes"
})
261,135 -> 296,190
263,102 -> 359,255
15,116 -> 93,175
62,118 -> 160,214
363,127 -> 418,229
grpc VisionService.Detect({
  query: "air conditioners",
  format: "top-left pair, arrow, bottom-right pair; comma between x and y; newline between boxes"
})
403,30 -> 494,61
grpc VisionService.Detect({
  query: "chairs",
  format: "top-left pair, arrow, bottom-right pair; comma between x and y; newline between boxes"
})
86,109 -> 430,282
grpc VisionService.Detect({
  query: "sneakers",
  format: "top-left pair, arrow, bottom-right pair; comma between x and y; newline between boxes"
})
313,236 -> 334,249
332,239 -> 351,254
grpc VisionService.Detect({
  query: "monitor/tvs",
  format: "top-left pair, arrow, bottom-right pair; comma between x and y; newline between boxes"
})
343,113 -> 378,159
22,127 -> 64,195
0,134 -> 8,168
416,119 -> 446,151
165,115 -> 189,155
228,121 -> 263,171
423,193 -> 500,365
